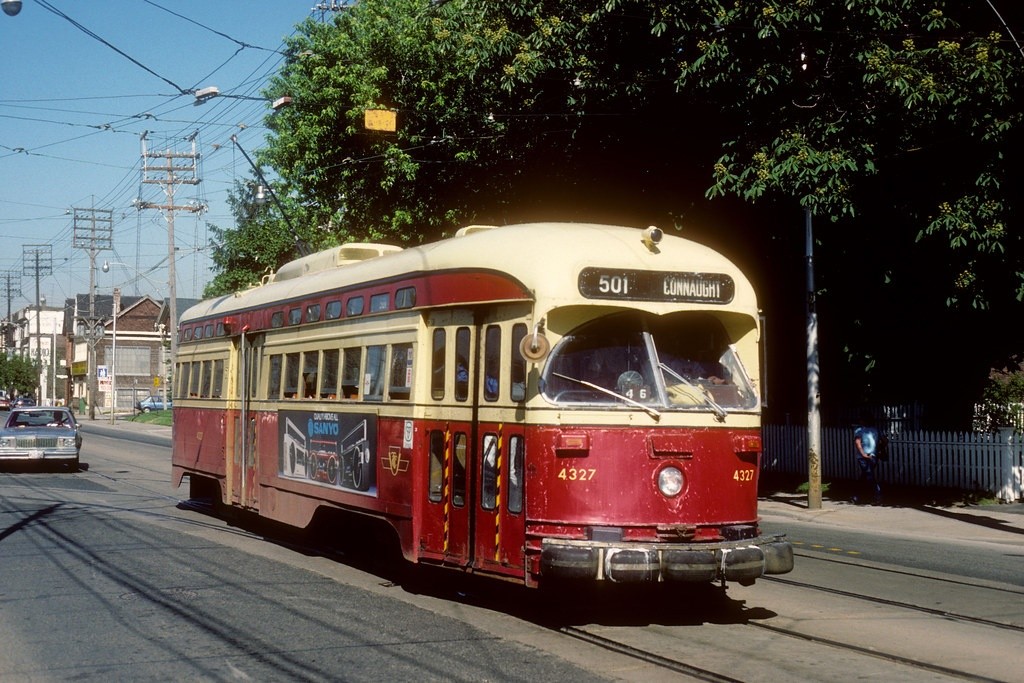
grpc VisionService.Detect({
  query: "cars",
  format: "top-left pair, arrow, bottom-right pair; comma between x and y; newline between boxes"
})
0,406 -> 83,473
6,398 -> 35,414
0,390 -> 11,410
134,395 -> 172,413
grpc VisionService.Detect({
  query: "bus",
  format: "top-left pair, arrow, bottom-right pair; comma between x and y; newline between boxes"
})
168,222 -> 797,594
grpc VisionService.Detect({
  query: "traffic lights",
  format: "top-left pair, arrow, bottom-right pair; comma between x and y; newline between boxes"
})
792,49 -> 820,108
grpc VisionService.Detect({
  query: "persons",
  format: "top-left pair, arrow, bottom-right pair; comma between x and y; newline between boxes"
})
483,436 -> 521,512
46,411 -> 72,428
851,414 -> 884,506
639,336 -> 725,388
429,430 -> 467,502
